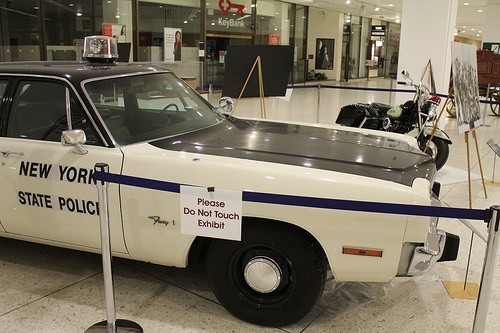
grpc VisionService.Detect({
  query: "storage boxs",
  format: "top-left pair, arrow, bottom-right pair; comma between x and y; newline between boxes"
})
477,49 -> 500,85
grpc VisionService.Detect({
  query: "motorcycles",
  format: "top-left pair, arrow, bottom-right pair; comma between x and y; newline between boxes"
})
335,69 -> 452,172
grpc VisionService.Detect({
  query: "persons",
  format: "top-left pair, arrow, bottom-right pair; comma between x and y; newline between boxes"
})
173,31 -> 181,61
119,26 -> 126,42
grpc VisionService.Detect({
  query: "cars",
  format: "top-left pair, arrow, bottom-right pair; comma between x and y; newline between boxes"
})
1,34 -> 446,328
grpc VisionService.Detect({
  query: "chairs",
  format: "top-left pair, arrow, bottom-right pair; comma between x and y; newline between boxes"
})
98,108 -> 131,144
